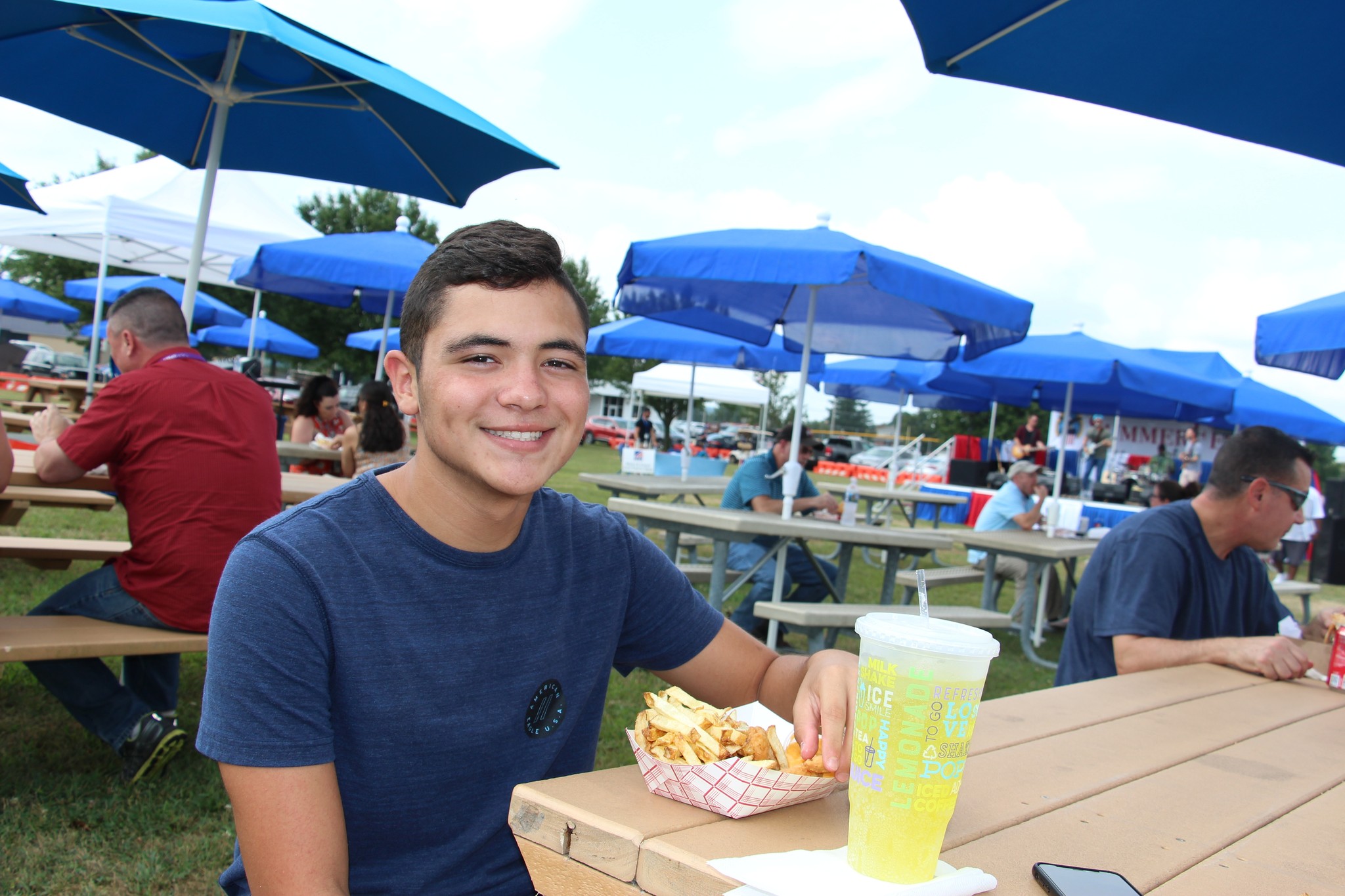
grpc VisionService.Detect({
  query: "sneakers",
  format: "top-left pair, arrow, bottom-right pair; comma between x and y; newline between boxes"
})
119,711 -> 187,788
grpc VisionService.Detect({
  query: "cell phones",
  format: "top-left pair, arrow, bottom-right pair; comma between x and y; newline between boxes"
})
1031,863 -> 1145,896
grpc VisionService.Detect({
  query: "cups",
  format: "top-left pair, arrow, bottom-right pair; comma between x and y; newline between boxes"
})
848,611 -> 1001,885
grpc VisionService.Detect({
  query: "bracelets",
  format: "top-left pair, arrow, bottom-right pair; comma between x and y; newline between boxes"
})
1184,454 -> 1189,461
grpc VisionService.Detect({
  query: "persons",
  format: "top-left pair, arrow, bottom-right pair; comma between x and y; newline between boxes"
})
288,375 -> 355,476
633,407 -> 658,449
1049,425 -> 1345,688
1077,413 -> 1112,501
1268,484 -> 1325,587
1177,426 -> 1203,488
329,381 -> 409,482
1014,413 -> 1047,464
28,287 -> 280,786
220,219 -> 861,896
1147,479 -> 1198,509
967,460 -> 1072,627
714,421 -> 838,646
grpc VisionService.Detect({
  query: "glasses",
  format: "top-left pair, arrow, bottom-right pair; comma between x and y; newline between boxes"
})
1238,475 -> 1308,511
801,446 -> 814,456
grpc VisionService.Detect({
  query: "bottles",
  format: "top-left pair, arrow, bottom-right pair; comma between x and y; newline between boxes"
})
839,476 -> 859,529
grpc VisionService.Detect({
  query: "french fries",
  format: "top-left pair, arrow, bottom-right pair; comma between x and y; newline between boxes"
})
634,685 -> 788,775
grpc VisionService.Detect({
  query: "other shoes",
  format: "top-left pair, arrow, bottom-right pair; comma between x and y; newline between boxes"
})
1272,570 -> 1290,583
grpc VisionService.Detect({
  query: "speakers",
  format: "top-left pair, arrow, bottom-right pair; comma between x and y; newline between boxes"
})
949,459 -> 1009,489
1092,482 -> 1126,505
1307,479 -> 1345,586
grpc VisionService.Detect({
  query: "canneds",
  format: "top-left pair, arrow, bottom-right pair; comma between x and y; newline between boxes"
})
1325,625 -> 1345,690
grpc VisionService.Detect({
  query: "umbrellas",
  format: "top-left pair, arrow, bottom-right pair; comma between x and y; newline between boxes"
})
0,0 -> 562,339
580,215 -> 1345,653
5,217 -> 443,382
902,0 -> 1345,167
0,161 -> 48,214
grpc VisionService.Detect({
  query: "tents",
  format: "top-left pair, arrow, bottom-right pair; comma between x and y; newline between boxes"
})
623,361 -> 833,452
4,155 -> 324,413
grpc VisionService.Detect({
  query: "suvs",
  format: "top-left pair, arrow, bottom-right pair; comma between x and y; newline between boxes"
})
8,339 -> 103,385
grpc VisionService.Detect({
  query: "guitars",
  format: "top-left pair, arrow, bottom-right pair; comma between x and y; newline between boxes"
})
1012,444 -> 1056,459
1082,436 -> 1112,459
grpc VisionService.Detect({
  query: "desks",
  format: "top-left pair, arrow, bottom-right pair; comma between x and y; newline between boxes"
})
0,376 -> 359,501
577,446 -> 1103,669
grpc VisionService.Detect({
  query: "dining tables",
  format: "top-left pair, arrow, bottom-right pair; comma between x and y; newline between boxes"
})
508,631 -> 1345,896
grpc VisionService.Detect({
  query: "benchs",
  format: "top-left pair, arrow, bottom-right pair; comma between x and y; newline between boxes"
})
0,403 -> 1322,663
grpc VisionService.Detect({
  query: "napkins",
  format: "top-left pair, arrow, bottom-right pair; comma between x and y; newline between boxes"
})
705,843 -> 997,896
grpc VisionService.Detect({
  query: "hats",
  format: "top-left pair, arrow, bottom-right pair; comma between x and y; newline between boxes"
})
775,423 -> 824,451
1006,460 -> 1043,480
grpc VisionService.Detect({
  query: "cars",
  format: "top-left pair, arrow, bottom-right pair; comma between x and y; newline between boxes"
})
848,445 -> 948,484
216,357 -> 368,413
823,436 -> 879,457
578,415 -> 778,459
